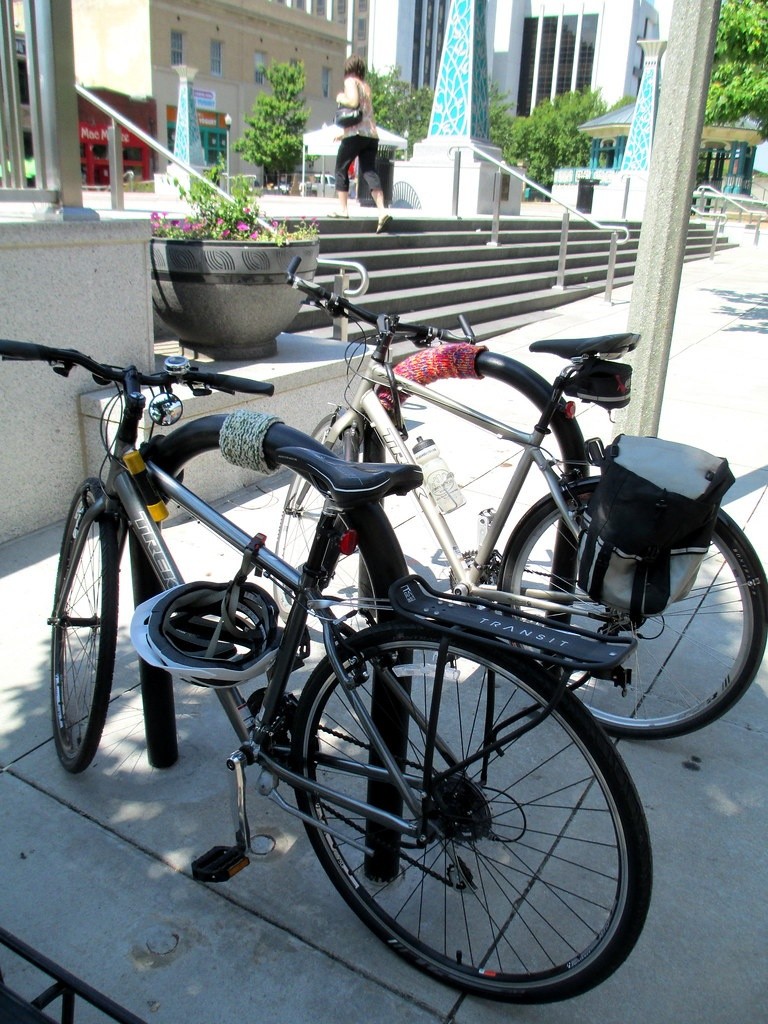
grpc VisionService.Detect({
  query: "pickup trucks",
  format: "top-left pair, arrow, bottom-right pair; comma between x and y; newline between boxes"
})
299,173 -> 336,196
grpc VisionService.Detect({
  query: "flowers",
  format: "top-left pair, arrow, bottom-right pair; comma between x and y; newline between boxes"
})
143,163 -> 323,244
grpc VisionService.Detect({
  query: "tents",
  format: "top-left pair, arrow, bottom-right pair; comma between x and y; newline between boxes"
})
302,123 -> 407,197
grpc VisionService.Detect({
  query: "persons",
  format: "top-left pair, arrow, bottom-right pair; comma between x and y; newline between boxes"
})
328,55 -> 393,235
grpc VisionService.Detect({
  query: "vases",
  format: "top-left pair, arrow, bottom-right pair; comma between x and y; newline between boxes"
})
150,238 -> 322,361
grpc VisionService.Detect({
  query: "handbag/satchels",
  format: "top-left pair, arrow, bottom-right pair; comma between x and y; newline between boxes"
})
335,103 -> 362,126
574,435 -> 735,622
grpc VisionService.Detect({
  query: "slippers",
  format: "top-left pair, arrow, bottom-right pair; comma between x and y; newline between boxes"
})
377,214 -> 393,232
329,211 -> 349,219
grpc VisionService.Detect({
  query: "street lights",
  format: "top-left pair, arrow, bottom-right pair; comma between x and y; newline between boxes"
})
319,122 -> 328,197
223,112 -> 232,196
403,129 -> 409,160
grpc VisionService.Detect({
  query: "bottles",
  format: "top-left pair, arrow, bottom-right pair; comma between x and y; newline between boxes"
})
414,435 -> 465,514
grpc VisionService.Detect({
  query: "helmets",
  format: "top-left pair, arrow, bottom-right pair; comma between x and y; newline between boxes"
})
129,577 -> 283,689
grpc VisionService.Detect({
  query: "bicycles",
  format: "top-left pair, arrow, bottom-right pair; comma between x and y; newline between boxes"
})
275,255 -> 768,741
0,329 -> 652,1006
